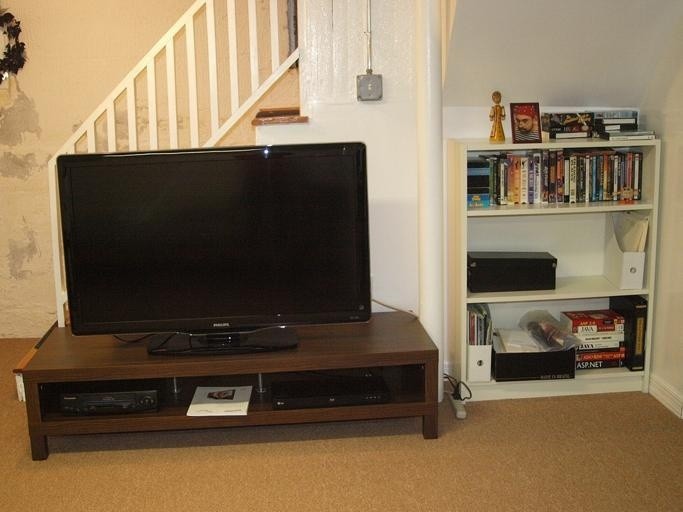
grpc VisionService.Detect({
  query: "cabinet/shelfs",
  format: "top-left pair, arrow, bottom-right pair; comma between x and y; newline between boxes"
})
447,136 -> 664,420
23,312 -> 440,464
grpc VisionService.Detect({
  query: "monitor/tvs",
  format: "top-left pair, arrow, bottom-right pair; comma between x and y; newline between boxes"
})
56,141 -> 371,355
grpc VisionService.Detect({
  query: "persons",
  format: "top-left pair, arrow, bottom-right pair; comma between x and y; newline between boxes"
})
487,90 -> 507,144
515,105 -> 539,140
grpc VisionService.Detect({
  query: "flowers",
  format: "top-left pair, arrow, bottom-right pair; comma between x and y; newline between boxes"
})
0,6 -> 28,87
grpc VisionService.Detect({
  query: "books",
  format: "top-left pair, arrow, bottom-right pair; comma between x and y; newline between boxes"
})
478,149 -> 642,205
466,298 -> 648,383
180,386 -> 255,419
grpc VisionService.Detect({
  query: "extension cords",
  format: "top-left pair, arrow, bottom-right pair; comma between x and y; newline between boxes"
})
448,393 -> 467,419
